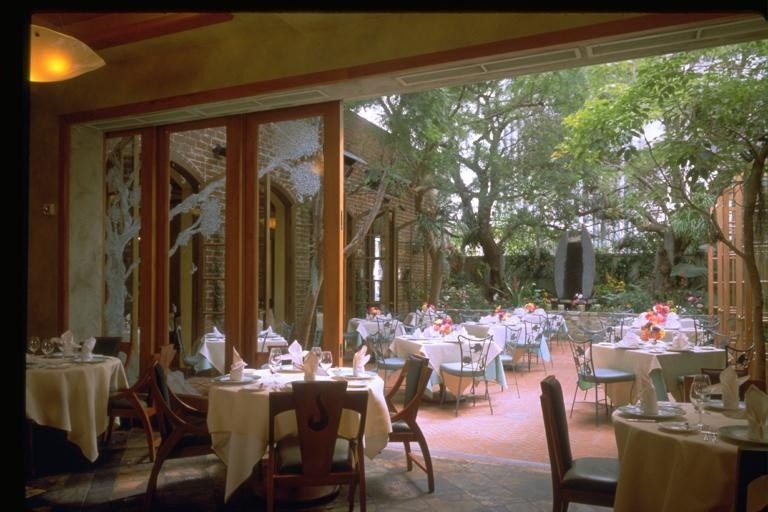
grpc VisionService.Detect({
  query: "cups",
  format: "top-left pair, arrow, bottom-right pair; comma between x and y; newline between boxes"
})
301,364 -> 318,381
230,363 -> 245,380
352,359 -> 365,376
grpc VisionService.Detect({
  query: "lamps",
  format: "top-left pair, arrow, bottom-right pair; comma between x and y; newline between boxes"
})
29,24 -> 107,84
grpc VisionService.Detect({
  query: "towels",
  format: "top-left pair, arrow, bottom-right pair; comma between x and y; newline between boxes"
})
229,346 -> 248,371
60,330 -> 81,351
261,325 -> 273,336
275,339 -> 309,365
296,350 -> 329,378
81,336 -> 96,355
213,326 -> 222,337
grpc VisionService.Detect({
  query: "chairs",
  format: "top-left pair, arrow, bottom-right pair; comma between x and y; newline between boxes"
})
107,344 -> 178,462
143,358 -> 217,505
264,380 -> 369,512
80,334 -> 134,371
268,345 -> 292,365
173,320 -> 211,377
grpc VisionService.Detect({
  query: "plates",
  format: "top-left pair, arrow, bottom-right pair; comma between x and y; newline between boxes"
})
336,371 -> 376,379
260,332 -> 282,341
657,421 -> 704,434
26,351 -> 110,369
616,404 -> 687,419
212,374 -> 262,384
597,342 -> 717,353
400,334 -> 465,345
718,424 -> 768,447
338,379 -> 369,387
205,332 -> 225,343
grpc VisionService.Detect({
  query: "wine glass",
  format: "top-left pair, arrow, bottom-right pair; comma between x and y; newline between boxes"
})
268,347 -> 282,372
689,375 -> 713,416
311,346 -> 322,366
29,336 -> 55,360
319,351 -> 332,373
